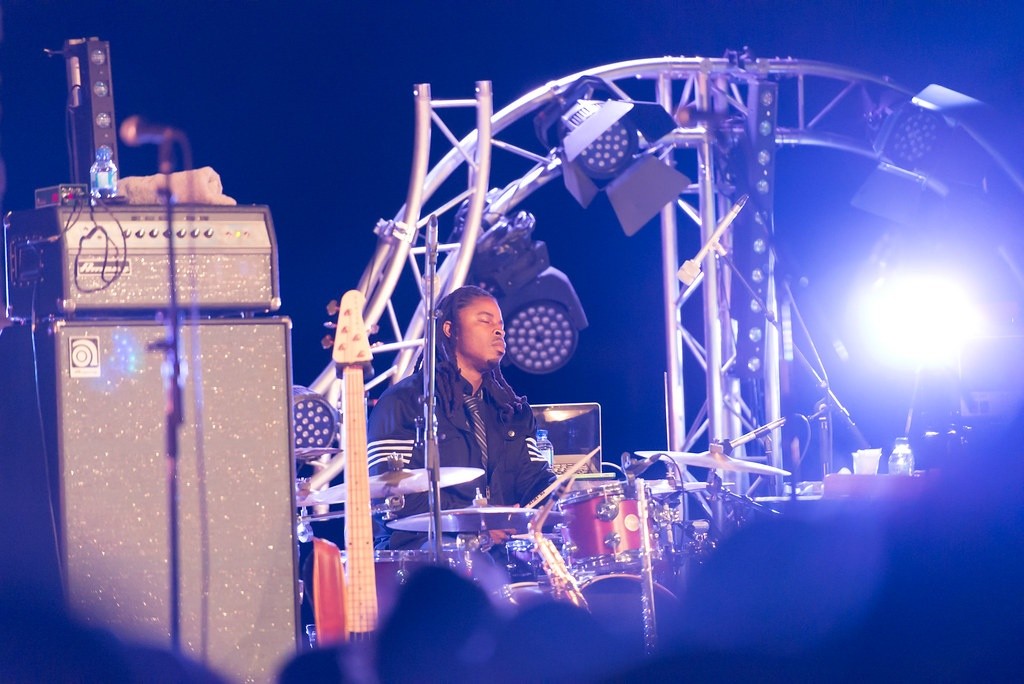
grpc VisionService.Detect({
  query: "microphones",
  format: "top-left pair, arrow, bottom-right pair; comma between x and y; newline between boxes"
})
691,194 -> 748,268
625,453 -> 662,476
534,473 -> 576,532
119,116 -> 176,147
731,412 -> 800,449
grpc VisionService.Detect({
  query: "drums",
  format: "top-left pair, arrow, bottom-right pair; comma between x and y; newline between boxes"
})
556,481 -> 670,580
490,581 -> 605,684
336,550 -> 463,642
504,536 -> 567,584
580,573 -> 690,683
667,541 -> 730,607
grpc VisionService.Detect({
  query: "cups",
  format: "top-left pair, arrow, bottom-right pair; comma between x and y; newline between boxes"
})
850,452 -> 882,475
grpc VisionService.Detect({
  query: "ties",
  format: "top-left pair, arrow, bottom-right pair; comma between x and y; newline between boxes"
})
462,391 -> 488,493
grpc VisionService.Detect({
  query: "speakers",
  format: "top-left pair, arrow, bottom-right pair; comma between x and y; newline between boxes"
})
6,316 -> 300,684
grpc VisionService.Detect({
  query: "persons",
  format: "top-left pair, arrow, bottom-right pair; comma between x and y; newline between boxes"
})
366,285 -> 570,559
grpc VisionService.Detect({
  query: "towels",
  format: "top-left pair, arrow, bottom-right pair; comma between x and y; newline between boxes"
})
117,166 -> 237,206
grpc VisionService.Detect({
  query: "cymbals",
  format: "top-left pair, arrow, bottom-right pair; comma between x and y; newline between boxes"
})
386,506 -> 578,534
311,465 -> 487,504
634,448 -> 792,477
646,478 -> 734,493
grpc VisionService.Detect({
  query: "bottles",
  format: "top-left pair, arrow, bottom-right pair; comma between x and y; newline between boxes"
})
90,147 -> 118,206
888,437 -> 915,476
536,430 -> 553,468
